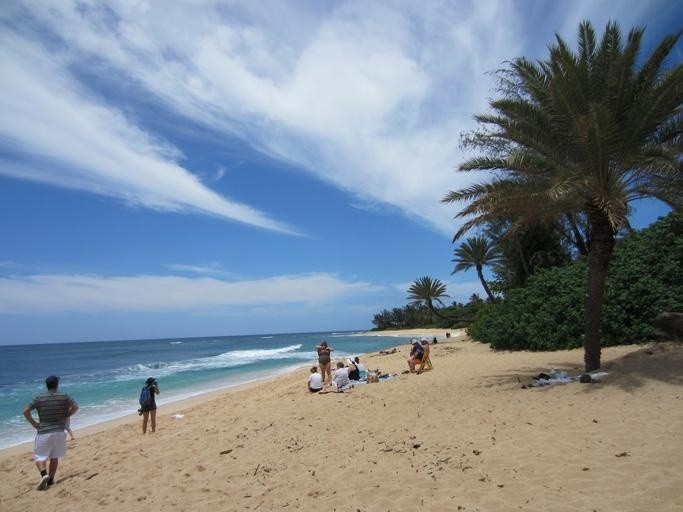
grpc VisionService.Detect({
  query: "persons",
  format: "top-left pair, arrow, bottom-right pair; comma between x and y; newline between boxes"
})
308,367 -> 323,393
332,361 -> 350,388
407,338 -> 425,373
315,341 -> 334,382
420,338 -> 429,360
23,376 -> 79,492
355,357 -> 366,377
139,377 -> 160,434
64,416 -> 75,440
348,361 -> 360,380
378,348 -> 397,355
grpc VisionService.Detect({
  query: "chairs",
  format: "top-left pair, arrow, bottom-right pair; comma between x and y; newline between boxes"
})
410,342 -> 433,374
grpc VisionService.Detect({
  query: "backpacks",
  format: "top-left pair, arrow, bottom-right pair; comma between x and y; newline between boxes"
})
139,386 -> 152,406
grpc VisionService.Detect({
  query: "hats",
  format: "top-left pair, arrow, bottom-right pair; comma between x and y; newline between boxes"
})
145,378 -> 155,383
421,338 -> 428,342
311,367 -> 317,372
348,358 -> 357,366
46,376 -> 58,387
411,339 -> 417,344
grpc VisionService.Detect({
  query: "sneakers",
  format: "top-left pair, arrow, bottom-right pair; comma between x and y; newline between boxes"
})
37,475 -> 50,490
